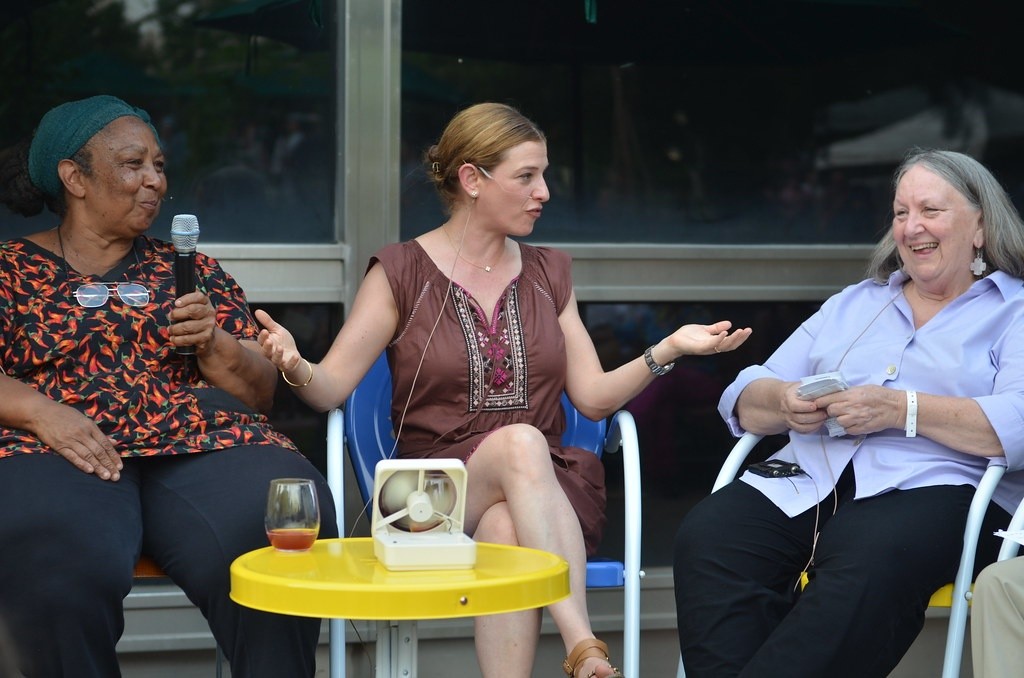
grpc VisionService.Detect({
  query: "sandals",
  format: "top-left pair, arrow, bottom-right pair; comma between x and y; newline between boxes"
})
562,639 -> 623,678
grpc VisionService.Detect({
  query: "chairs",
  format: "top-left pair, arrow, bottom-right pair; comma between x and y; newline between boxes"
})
328,355 -> 642,678
678,424 -> 1024,678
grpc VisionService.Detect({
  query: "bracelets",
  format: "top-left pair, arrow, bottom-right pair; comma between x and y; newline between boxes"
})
281,358 -> 313,387
904,390 -> 918,439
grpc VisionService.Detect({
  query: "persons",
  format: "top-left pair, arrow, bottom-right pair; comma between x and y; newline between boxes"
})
0,96 -> 341,678
971,556 -> 1024,678
254,103 -> 751,678
672,149 -> 1024,678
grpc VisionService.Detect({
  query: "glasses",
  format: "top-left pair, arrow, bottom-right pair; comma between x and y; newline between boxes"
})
64,283 -> 152,307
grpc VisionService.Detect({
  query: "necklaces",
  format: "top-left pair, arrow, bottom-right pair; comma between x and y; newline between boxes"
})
443,225 -> 506,271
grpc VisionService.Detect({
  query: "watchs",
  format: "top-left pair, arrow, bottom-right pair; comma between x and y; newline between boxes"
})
644,344 -> 675,375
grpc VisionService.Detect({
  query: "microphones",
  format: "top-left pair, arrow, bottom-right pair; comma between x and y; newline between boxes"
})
493,180 -> 532,199
172,215 -> 199,355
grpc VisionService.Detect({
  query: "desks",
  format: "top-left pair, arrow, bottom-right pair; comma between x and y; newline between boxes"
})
230,539 -> 571,678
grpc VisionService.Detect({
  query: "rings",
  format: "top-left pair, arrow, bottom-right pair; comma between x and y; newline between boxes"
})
714,347 -> 721,353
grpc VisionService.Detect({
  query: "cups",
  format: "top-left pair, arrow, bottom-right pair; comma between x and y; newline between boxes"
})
264,477 -> 321,553
